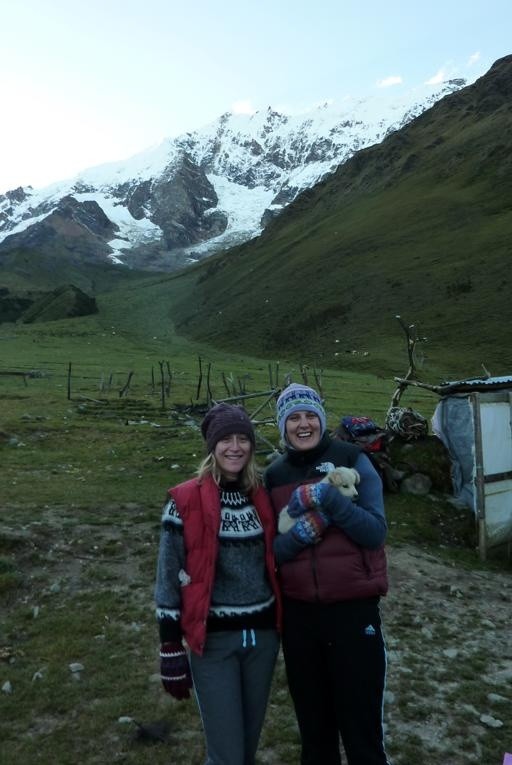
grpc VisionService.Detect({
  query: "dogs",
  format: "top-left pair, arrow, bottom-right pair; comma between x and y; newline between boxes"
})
275,466 -> 362,535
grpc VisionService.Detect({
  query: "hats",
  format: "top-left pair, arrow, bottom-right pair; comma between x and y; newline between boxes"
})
201,403 -> 256,454
277,383 -> 326,450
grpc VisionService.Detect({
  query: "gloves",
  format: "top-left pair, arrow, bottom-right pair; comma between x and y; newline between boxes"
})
286,484 -> 322,518
160,642 -> 194,701
293,508 -> 331,544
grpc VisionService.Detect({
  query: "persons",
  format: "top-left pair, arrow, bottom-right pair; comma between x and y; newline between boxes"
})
153,403 -> 282,765
263,383 -> 389,765
